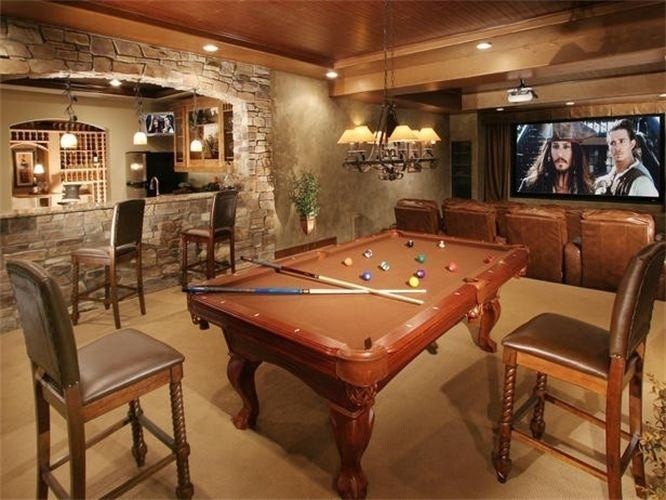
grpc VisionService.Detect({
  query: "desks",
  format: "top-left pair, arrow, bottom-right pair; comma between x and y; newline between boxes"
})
187,230 -> 531,500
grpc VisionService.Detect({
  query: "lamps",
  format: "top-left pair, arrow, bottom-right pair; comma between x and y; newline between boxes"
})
338,0 -> 443,183
188,91 -> 204,153
130,82 -> 149,146
506,79 -> 533,103
58,77 -> 80,150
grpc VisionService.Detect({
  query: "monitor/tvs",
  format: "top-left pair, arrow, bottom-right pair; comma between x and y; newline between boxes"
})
511,113 -> 662,203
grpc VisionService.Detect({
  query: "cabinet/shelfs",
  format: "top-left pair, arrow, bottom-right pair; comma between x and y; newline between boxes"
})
173,100 -> 233,172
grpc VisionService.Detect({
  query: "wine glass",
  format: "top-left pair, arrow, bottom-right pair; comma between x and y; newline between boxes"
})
38,181 -> 48,195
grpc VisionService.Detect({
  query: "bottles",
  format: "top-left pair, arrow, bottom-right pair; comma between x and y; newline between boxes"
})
32,177 -> 38,195
223,165 -> 235,188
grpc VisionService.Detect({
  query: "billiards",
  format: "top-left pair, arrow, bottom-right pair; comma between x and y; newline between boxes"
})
468,308 -> 483,322
448,264 -> 456,271
438,239 -> 446,248
486,254 -> 496,264
344,250 -> 391,281
408,239 -> 414,248
409,254 -> 428,288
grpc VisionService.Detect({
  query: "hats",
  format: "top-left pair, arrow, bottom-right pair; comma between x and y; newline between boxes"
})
542,121 -> 596,143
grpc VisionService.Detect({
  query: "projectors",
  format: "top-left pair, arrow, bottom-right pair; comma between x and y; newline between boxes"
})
506,88 -> 538,103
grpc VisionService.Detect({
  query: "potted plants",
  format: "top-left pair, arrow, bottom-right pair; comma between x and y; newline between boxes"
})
288,170 -> 319,234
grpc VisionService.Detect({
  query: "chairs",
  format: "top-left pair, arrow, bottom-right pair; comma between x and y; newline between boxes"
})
6,260 -> 193,499
180,189 -> 236,284
493,242 -> 666,500
70,198 -> 145,329
393,198 -> 666,300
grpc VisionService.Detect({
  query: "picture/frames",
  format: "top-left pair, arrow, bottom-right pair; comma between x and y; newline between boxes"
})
12,148 -> 35,187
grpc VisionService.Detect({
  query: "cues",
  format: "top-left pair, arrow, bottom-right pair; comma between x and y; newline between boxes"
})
240,253 -> 424,309
182,283 -> 428,296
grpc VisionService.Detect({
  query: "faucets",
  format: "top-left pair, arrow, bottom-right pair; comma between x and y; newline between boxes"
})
150,176 -> 160,196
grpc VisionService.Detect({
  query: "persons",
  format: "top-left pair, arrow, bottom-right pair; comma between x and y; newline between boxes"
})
147,114 -> 174,133
518,118 -> 595,198
593,119 -> 659,197
